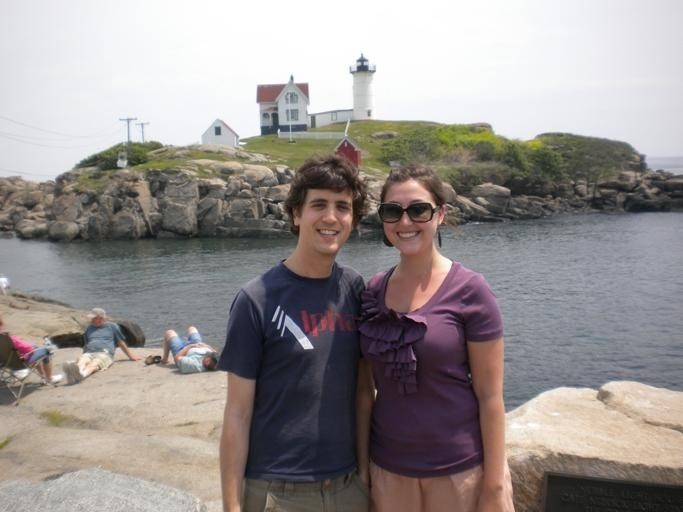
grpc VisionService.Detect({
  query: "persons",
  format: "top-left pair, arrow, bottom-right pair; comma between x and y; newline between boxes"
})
213,151 -> 375,511
349,162 -> 515,512
0,317 -> 63,387
60,306 -> 142,385
158,324 -> 219,375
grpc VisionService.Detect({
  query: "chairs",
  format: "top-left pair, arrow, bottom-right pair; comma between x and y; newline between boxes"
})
0,329 -> 59,408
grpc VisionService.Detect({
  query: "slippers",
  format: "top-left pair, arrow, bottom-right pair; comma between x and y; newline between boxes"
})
153,355 -> 162,363
145,355 -> 154,365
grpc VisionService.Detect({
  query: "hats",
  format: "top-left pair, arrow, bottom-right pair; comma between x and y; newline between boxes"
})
86,307 -> 106,319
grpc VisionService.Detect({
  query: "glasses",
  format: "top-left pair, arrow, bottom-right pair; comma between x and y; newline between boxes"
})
377,203 -> 441,224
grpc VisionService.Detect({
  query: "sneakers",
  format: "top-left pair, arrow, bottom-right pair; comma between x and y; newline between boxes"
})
62,359 -> 76,385
69,360 -> 85,382
41,374 -> 63,385
